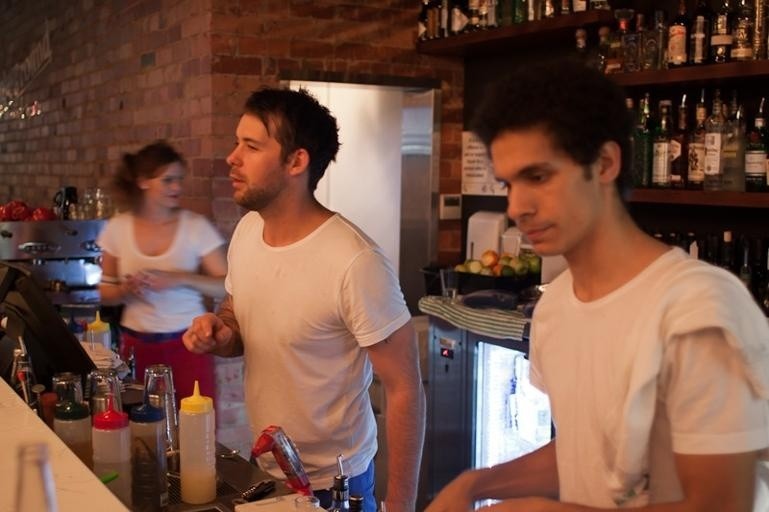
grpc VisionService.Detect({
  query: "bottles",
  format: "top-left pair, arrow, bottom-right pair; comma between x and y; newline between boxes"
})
53,382 -> 217,512
416,0 -> 769,69
623,88 -> 769,314
67,310 -> 111,356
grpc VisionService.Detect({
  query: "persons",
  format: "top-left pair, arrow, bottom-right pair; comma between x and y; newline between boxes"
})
180,85 -> 427,512
92,138 -> 229,440
424,60 -> 769,511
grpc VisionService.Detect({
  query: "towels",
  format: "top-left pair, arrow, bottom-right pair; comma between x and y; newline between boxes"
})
591,259 -> 768,505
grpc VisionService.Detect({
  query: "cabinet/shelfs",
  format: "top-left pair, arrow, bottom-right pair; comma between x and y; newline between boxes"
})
415,0 -> 769,317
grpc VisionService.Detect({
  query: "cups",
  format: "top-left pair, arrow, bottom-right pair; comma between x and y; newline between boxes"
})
53,186 -> 113,220
52,364 -> 177,451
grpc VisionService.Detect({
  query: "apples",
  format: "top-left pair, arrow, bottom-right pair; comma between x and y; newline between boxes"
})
453,250 -> 541,276
0,201 -> 57,222
